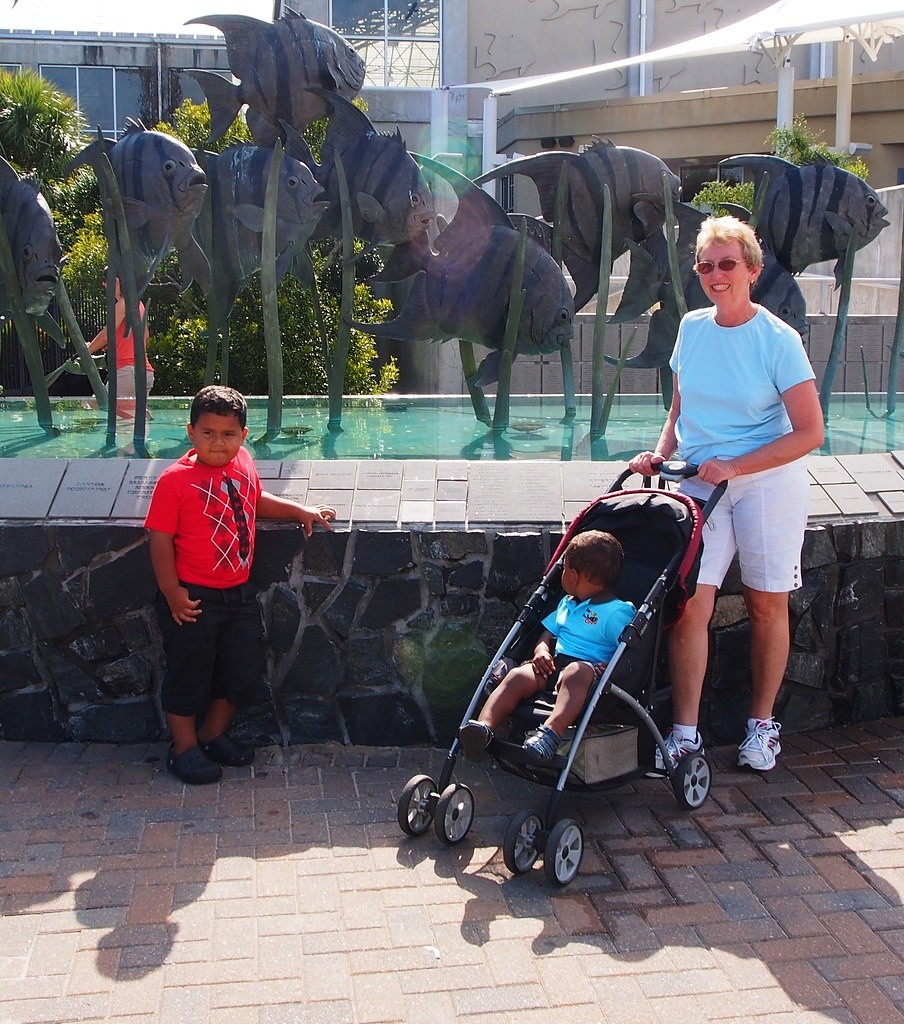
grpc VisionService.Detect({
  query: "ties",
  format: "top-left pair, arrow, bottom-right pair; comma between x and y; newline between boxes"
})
223,475 -> 249,562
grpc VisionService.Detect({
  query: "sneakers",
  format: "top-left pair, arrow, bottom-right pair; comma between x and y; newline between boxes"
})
522,724 -> 562,762
737,716 -> 782,773
643,728 -> 704,778
456,719 -> 494,760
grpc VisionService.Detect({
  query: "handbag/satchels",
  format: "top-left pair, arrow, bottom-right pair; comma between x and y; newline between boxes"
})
524,721 -> 638,784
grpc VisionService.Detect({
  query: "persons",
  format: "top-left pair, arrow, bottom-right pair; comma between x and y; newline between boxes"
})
144,385 -> 335,784
459,530 -> 638,763
64,266 -> 155,397
629,213 -> 825,777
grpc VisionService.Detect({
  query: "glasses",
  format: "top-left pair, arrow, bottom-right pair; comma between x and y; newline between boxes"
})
694,258 -> 748,275
102,278 -> 107,283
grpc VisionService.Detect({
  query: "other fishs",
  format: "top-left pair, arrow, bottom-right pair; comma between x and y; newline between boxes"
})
181,4 -> 366,150
341,150 -> 576,387
179,135 -> 331,295
277,86 -> 436,268
0,157 -> 67,349
603,192 -> 810,370
716,151 -> 891,291
471,134 -> 685,313
62,116 -> 213,301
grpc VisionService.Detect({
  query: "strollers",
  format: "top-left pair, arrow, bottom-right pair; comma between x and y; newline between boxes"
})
397,456 -> 728,890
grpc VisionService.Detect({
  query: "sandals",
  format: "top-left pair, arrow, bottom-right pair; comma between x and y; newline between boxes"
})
166,741 -> 223,784
194,730 -> 256,767
